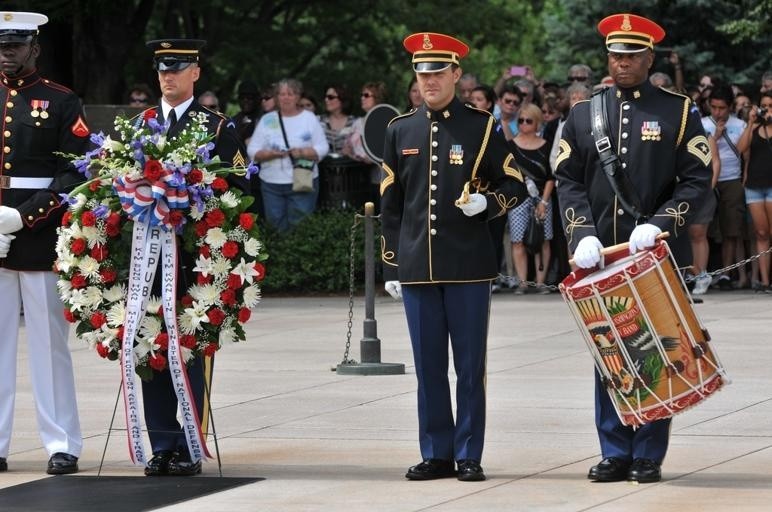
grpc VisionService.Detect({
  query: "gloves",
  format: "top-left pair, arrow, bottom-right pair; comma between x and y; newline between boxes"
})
573,235 -> 604,269
385,281 -> 403,296
628,222 -> 663,252
0,203 -> 22,233
460,194 -> 488,216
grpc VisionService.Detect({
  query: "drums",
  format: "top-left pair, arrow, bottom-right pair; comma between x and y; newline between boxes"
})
559,238 -> 731,427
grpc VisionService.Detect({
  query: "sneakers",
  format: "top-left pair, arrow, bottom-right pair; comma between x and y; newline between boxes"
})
692,275 -> 713,295
716,273 -> 733,291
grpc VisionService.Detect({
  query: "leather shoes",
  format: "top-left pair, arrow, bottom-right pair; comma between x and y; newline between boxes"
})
407,458 -> 486,480
144,452 -> 204,476
588,456 -> 663,481
46,453 -> 80,473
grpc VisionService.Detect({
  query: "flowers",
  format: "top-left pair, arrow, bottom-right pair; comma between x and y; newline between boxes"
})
46,102 -> 274,380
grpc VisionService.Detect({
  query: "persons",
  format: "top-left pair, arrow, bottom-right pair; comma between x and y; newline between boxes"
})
0,9 -> 94,479
550,12 -> 715,486
118,36 -> 264,479
376,31 -> 529,484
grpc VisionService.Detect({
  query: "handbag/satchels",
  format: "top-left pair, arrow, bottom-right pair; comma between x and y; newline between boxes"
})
522,211 -> 545,255
292,155 -> 315,192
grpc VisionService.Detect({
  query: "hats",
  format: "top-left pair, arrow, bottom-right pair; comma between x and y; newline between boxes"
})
402,30 -> 470,71
597,11 -> 667,52
146,37 -> 209,71
0,11 -> 49,45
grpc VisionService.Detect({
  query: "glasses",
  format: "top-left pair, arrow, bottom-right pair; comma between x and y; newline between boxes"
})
501,83 -> 554,124
324,89 -> 337,102
361,91 -> 371,98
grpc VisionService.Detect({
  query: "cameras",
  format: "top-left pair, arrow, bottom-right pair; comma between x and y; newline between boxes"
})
511,66 -> 527,75
757,108 -> 768,116
